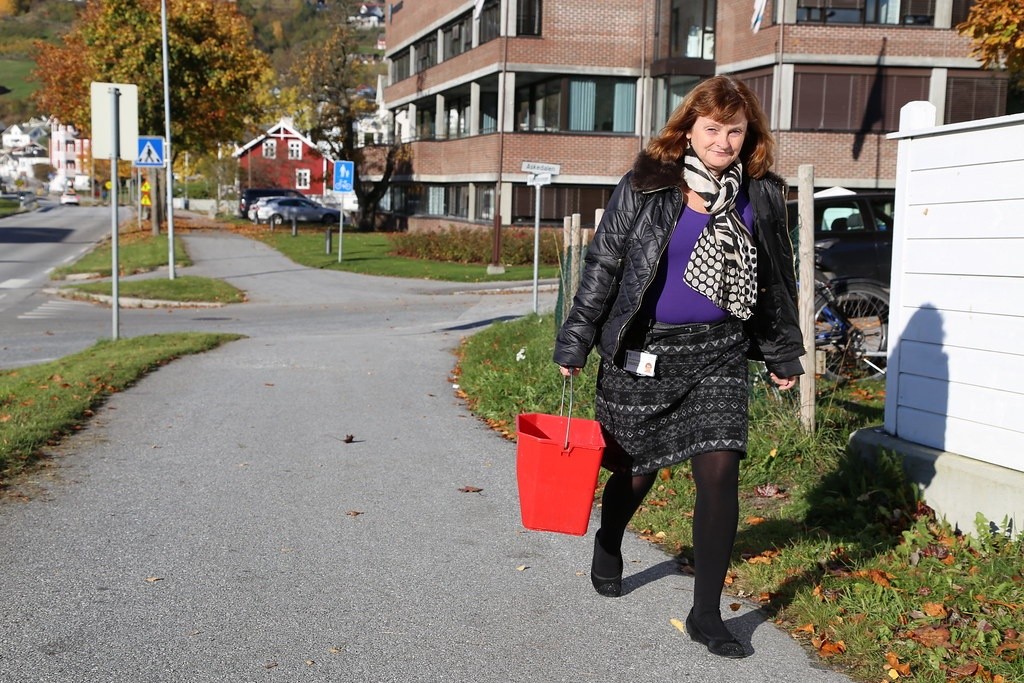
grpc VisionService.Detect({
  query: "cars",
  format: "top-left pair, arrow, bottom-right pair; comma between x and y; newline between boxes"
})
59,192 -> 80,205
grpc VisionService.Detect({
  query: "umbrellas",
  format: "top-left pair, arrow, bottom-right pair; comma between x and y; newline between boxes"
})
814,186 -> 858,197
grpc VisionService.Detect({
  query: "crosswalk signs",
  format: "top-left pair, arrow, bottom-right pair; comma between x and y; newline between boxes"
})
134,134 -> 164,165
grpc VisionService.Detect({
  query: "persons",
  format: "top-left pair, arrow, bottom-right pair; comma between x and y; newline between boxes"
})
552,75 -> 806,658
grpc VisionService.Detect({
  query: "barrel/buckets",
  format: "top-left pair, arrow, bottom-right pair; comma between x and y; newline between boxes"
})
515,366 -> 606,536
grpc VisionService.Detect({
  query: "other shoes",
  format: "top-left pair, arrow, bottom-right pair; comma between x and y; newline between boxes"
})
686,606 -> 747,658
590,528 -> 624,597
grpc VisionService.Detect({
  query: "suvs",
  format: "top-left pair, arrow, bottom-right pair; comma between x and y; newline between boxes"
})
786,191 -> 895,319
239,187 -> 345,226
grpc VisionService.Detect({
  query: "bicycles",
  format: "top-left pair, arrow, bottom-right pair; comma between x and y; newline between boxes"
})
813,268 -> 889,383
